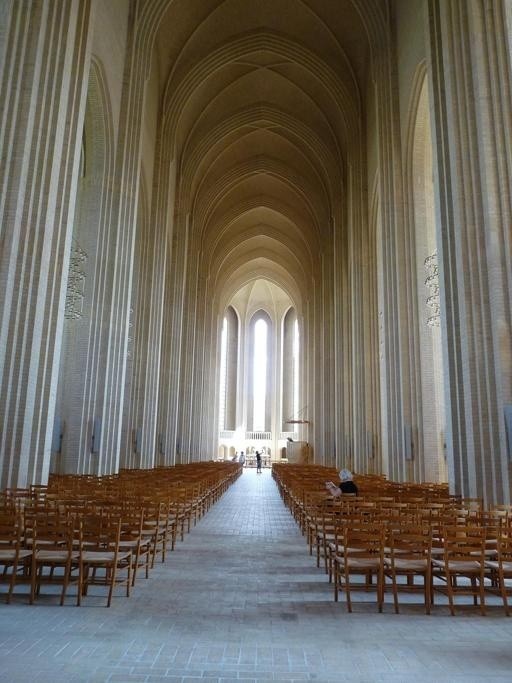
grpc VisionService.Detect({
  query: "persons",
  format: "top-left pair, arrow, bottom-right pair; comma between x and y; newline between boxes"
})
318,468 -> 357,514
239,450 -> 245,463
234,451 -> 238,458
256,450 -> 263,473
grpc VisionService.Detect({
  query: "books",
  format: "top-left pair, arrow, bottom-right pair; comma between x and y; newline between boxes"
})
326,480 -> 336,488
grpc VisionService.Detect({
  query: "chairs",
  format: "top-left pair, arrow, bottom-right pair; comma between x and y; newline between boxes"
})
271,462 -> 511,618
1,460 -> 244,608
